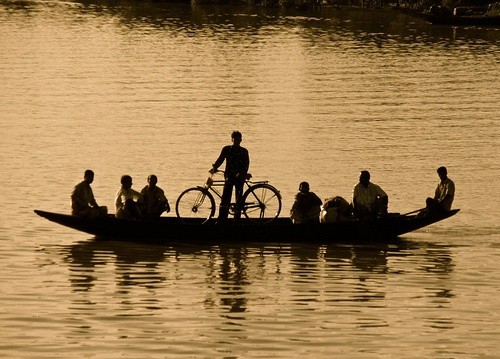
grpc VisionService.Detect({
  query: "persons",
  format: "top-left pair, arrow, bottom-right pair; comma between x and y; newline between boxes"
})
71,170 -> 107,218
137,174 -> 170,221
115,175 -> 143,221
211,130 -> 250,225
290,182 -> 322,225
415,166 -> 455,219
353,171 -> 388,219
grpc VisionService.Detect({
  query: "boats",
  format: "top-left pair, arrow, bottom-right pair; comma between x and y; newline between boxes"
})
34,209 -> 461,244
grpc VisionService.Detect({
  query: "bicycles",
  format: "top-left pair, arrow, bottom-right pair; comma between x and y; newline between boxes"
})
175,168 -> 282,224
